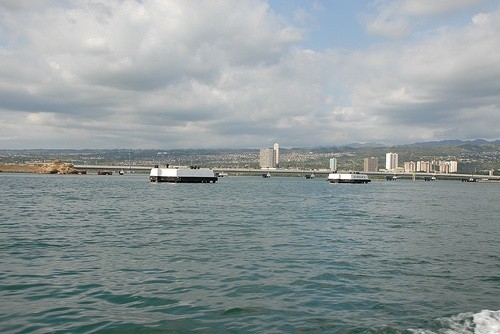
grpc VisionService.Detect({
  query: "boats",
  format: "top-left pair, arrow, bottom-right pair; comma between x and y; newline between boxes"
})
147,164 -> 220,185
326,170 -> 372,186
